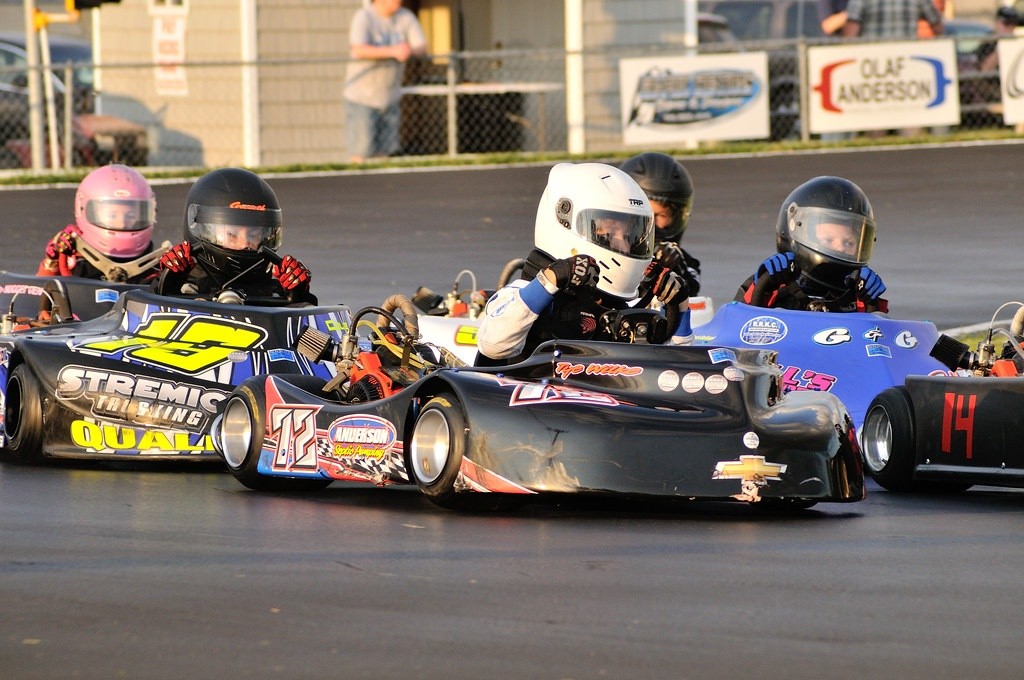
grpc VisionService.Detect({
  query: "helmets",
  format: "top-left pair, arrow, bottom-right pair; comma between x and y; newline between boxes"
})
74,164 -> 156,259
618,152 -> 695,248
183,168 -> 284,284
533,162 -> 656,300
775,175 -> 876,292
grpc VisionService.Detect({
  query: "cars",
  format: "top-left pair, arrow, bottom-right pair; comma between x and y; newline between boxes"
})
0,31 -> 93,150
0,82 -> 150,170
939,18 -> 1007,129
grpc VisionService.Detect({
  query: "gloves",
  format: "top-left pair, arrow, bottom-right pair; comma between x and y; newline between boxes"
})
45,224 -> 83,270
550,254 -> 600,290
754,251 -> 799,294
160,241 -> 197,284
645,262 -> 690,306
272,255 -> 313,294
845,266 -> 886,304
654,242 -> 687,276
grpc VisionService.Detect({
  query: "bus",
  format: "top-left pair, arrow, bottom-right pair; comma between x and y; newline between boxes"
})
695,13 -> 801,141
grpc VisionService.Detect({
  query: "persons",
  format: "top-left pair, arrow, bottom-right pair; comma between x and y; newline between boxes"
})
733,175 -> 886,313
474,152 -> 700,378
159,168 -> 318,307
814,0 -> 1024,141
36,164 -> 156,284
344,0 -> 427,161
